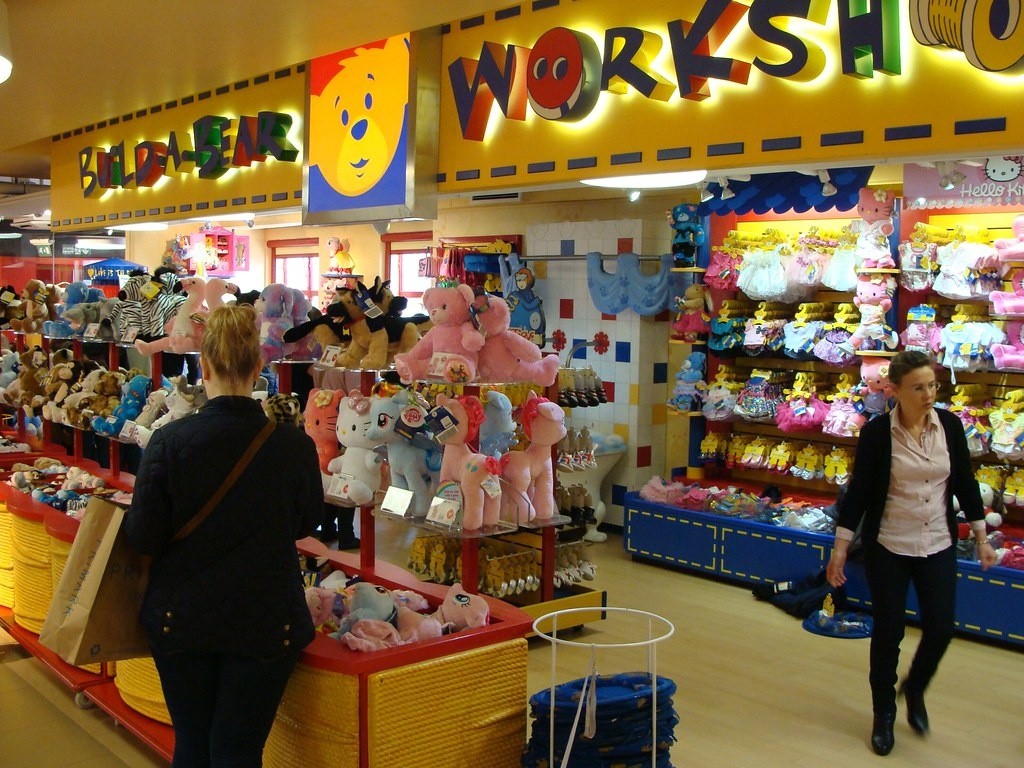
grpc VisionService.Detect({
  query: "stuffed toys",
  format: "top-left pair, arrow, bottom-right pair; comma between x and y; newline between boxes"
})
952,479 -> 1002,540
669,202 -> 706,268
1,237 -> 567,653
841,187 -> 900,420
668,352 -> 706,411
674,282 -> 717,340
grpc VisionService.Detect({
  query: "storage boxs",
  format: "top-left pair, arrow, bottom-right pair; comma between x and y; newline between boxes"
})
624,492 -> 1024,647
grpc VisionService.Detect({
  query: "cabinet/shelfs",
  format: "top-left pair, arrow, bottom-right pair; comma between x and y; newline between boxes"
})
0,328 -> 606,768
666,266 -> 707,416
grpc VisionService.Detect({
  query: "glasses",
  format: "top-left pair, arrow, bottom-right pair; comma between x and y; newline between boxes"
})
898,379 -> 941,394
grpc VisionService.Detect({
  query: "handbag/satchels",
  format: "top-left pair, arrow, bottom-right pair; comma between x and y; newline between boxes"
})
37,495 -> 153,666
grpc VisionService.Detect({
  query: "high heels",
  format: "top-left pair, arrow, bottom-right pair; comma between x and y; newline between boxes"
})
871,705 -> 896,755
900,676 -> 929,734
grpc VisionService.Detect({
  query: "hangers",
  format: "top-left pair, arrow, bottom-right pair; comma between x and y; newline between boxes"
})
701,222 -> 1024,499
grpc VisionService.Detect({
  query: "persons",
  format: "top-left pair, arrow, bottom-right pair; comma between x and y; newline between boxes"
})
118,303 -> 326,768
825,351 -> 997,757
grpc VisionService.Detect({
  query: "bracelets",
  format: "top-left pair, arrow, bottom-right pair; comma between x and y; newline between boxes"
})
975,539 -> 991,544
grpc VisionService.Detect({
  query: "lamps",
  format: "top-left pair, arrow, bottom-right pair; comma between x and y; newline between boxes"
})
625,188 -> 640,202
718,177 -> 738,201
819,169 -> 838,197
938,162 -> 954,190
695,182 -> 715,202
